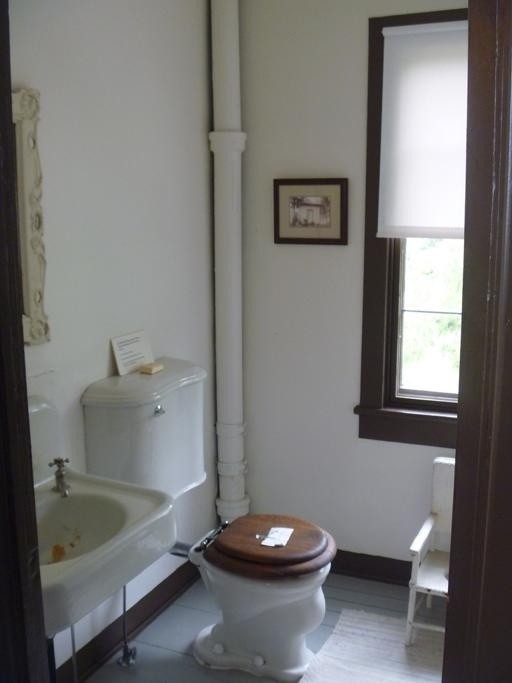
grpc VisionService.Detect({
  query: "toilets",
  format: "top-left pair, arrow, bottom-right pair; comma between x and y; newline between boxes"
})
78,357 -> 337,677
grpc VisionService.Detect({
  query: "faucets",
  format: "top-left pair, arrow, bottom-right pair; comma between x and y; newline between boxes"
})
49,455 -> 73,498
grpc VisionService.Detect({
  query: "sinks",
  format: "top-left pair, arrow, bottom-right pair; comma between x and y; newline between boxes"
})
34,471 -> 178,636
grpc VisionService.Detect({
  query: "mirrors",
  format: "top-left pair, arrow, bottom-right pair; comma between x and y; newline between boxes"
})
11,89 -> 50,345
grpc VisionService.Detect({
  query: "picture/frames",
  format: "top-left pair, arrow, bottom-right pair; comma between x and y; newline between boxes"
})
274,178 -> 348,245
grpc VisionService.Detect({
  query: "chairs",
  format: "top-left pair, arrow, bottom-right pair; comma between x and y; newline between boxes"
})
406,457 -> 456,646
